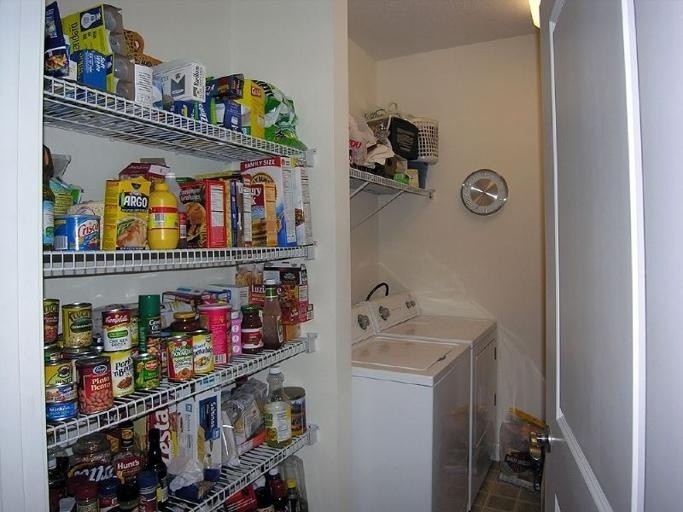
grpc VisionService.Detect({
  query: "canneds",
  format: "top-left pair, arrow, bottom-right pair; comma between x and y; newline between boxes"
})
273,387 -> 307,437
265,403 -> 292,449
43,298 -> 214,421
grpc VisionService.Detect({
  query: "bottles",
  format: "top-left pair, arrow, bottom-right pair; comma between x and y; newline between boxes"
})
146,182 -> 177,249
164,171 -> 186,246
136,294 -> 162,380
241,303 -> 263,351
170,311 -> 198,336
48,426 -> 167,511
264,279 -> 283,348
264,365 -> 292,447
229,309 -> 242,356
43,169 -> 55,250
258,469 -> 301,511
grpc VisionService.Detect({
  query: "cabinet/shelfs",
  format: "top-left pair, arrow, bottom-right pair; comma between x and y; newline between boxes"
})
35,73 -> 318,512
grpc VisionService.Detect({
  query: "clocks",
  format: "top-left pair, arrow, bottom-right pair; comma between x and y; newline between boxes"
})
459,169 -> 509,216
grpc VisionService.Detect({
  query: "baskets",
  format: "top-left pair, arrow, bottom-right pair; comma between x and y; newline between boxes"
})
410,116 -> 439,164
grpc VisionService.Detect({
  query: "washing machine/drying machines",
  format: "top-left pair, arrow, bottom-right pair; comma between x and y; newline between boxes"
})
369,292 -> 498,510
352,302 -> 472,510
